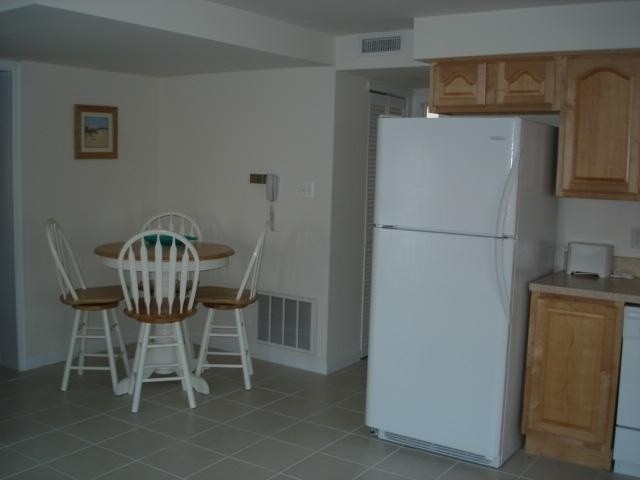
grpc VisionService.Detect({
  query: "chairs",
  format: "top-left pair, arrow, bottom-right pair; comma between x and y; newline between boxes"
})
136,212 -> 202,296
194,220 -> 268,390
45,218 -> 130,393
118,230 -> 199,413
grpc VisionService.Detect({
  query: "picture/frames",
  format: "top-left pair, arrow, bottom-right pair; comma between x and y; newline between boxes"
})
73,103 -> 117,160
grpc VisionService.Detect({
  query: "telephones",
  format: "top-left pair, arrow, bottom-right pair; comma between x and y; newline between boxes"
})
266,174 -> 278,201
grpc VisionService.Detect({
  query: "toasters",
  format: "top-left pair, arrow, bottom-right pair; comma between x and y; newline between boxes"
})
564,242 -> 614,279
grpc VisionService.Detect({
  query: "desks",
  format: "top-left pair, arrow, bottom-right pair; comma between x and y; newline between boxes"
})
95,239 -> 235,395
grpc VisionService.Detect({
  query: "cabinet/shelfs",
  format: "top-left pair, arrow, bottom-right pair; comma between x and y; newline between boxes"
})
528,297 -> 618,443
434,57 -> 553,106
561,56 -> 640,195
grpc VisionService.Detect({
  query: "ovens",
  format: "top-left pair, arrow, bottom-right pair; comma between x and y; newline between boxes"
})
617,308 -> 640,432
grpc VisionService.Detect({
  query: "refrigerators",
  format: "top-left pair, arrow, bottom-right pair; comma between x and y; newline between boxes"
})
361,113 -> 557,469
